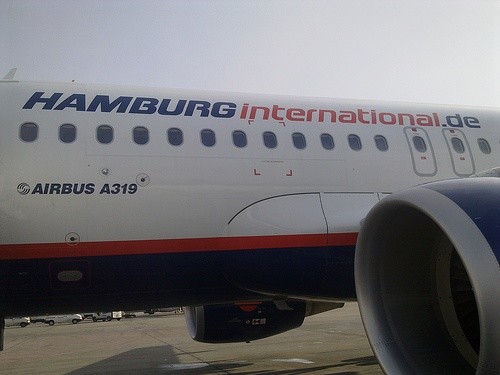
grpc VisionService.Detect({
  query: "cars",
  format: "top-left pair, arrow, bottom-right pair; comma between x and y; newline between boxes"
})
45,314 -> 82,326
92,311 -> 111,322
30,316 -> 45,323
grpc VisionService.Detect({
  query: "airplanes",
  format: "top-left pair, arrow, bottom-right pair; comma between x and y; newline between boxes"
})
0,68 -> 500,375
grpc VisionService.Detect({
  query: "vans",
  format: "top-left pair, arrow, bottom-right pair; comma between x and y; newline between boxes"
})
4,317 -> 30,327
